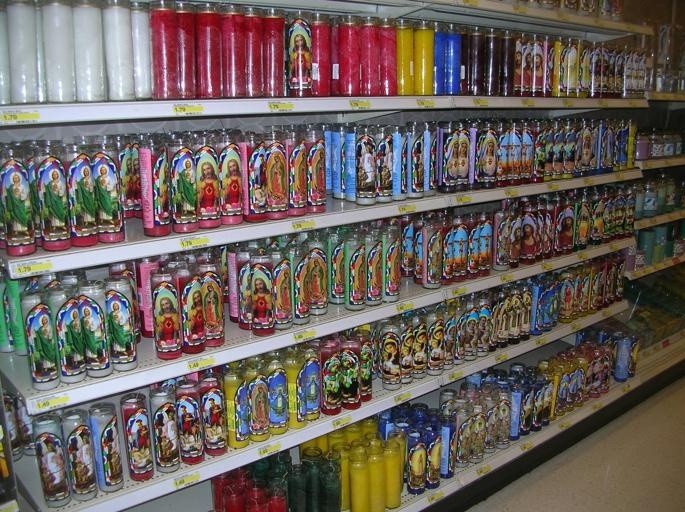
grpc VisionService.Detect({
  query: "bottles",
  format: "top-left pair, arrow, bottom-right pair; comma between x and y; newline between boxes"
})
0,230 -> 328,391
636,177 -> 677,220
177,323 -> 377,466
30,414 -> 70,508
328,185 -> 636,310
149,386 -> 181,473
377,251 -> 627,390
636,224 -> 684,274
439,328 -> 640,480
89,402 -> 124,493
120,394 -> 154,482
0,122 -> 331,255
211,401 -> 442,511
331,14 -> 646,99
636,127 -> 683,159
0,0 -> 331,105
331,118 -> 636,205
5,396 -> 36,463
61,409 -> 98,501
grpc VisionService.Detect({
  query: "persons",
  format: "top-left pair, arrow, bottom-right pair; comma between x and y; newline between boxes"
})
513,51 -> 544,89
290,33 -> 312,84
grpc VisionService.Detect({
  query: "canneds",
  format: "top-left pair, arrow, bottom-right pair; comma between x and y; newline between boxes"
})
633,172 -> 678,221
635,127 -> 683,161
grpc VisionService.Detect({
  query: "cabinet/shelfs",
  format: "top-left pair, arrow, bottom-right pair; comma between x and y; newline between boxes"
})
0,0 -> 685,512
